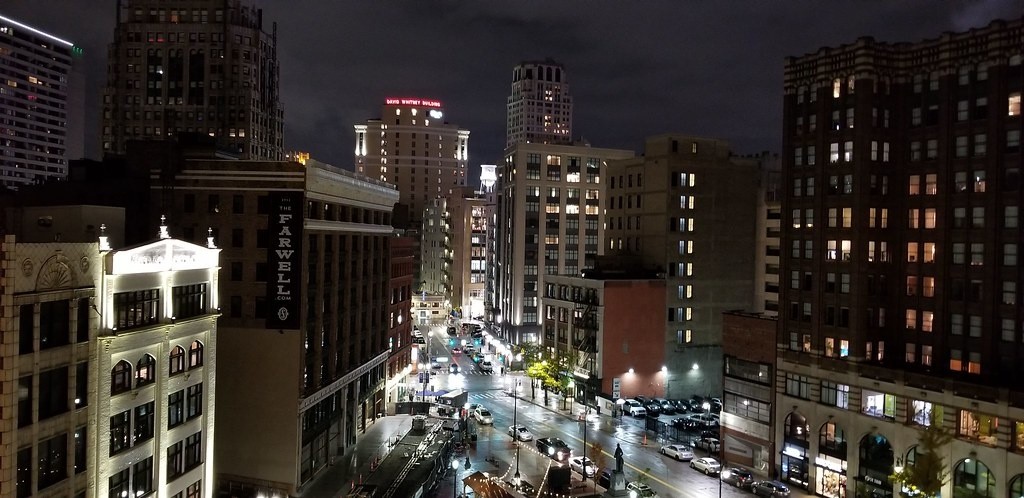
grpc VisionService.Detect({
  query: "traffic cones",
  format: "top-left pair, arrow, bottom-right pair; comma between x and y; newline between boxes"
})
642,435 -> 647,445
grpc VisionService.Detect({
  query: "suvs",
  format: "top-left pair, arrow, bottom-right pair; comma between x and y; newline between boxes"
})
752,481 -> 791,498
598,467 -> 629,488
721,467 -> 754,488
473,408 -> 494,425
627,481 -> 660,498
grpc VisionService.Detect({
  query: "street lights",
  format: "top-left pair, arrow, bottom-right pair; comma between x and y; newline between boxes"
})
513,379 -> 522,441
451,460 -> 459,498
582,406 -> 593,482
418,362 -> 432,401
427,327 -> 434,364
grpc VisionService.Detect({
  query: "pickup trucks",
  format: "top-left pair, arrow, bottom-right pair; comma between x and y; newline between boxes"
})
536,437 -> 571,459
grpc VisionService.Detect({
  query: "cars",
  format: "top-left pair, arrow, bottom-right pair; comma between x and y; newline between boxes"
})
619,395 -> 721,438
467,403 -> 484,417
431,355 -> 441,369
410,325 -> 425,344
569,456 -> 599,477
448,363 -> 461,372
462,341 -> 492,371
452,347 -> 461,355
690,457 -> 721,475
508,424 -> 533,442
661,444 -> 694,461
473,314 -> 484,321
695,438 -> 720,453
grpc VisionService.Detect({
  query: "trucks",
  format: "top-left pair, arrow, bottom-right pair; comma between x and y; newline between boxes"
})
435,389 -> 468,416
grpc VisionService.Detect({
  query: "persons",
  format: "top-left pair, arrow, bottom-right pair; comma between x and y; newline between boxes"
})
614,443 -> 623,472
